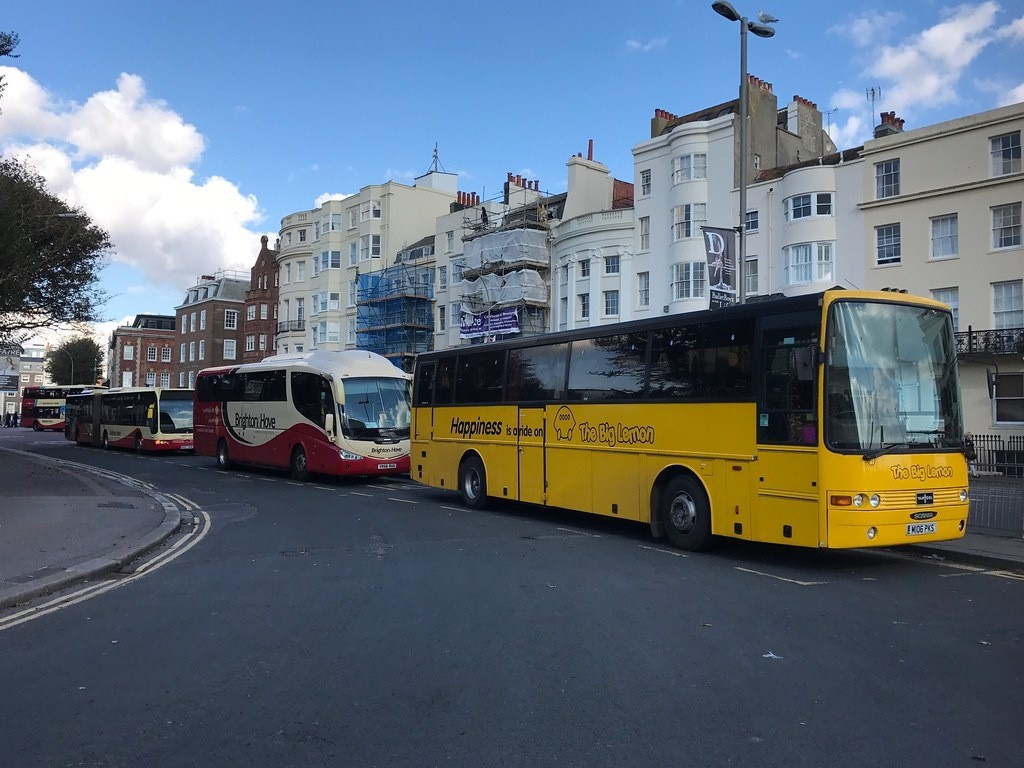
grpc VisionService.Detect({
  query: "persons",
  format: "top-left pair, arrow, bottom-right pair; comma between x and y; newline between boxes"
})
12,412 -> 19,427
964,432 -> 980,478
6,412 -> 11,427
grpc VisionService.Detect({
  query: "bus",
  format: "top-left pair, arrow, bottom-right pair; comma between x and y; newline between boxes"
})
409,289 -> 1000,552
65,387 -> 195,455
20,385 -> 109,431
193,350 -> 414,482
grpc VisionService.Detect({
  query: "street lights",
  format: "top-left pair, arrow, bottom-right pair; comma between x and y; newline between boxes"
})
51,346 -> 73,385
711,0 -> 776,304
